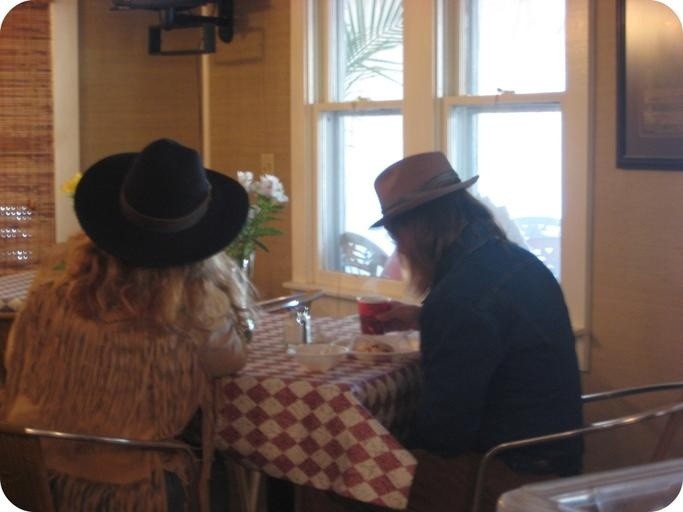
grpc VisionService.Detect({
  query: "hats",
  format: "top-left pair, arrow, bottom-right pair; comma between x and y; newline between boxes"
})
74,138 -> 250,268
369,152 -> 479,228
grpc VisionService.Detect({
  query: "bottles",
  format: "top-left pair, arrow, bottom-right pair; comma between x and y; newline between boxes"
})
301,306 -> 312,344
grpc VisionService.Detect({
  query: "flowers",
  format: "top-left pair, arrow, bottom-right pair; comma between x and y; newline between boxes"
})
226,170 -> 290,266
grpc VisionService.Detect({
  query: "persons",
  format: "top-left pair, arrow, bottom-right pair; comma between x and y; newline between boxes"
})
372,151 -> 584,511
1,136 -> 250,511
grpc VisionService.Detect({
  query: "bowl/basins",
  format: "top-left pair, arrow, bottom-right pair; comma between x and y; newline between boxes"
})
287,343 -> 348,370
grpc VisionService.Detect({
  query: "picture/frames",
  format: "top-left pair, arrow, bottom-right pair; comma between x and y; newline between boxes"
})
615,0 -> 683,169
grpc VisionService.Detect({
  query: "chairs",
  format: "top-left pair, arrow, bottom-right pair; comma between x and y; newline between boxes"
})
0,424 -> 204,512
248,287 -> 325,315
471,383 -> 682,512
340,231 -> 388,277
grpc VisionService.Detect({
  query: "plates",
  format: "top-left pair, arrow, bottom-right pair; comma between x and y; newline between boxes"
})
331,334 -> 420,363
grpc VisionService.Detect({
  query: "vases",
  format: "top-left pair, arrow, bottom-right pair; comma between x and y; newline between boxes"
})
237,259 -> 252,303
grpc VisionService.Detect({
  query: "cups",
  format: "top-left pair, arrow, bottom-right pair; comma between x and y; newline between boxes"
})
356,295 -> 392,335
